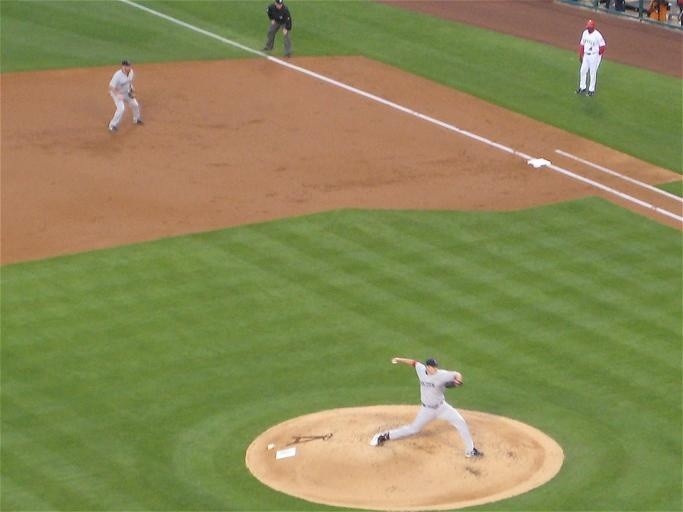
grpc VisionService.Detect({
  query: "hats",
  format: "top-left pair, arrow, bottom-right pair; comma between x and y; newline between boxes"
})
425,359 -> 440,366
585,20 -> 596,30
121,60 -> 131,65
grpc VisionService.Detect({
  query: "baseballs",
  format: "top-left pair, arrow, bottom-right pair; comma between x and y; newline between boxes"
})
392,360 -> 398,365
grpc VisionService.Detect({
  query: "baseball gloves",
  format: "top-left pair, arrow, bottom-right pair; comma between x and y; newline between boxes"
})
445,382 -> 457,388
128,90 -> 136,98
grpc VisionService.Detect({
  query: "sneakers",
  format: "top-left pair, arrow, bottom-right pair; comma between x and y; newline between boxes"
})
109,125 -> 115,132
574,88 -> 596,97
373,431 -> 389,445
464,448 -> 483,459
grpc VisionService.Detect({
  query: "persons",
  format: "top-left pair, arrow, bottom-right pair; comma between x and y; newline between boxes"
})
573,19 -> 607,97
260,1 -> 294,60
597,1 -> 682,28
107,58 -> 144,134
369,356 -> 488,459
